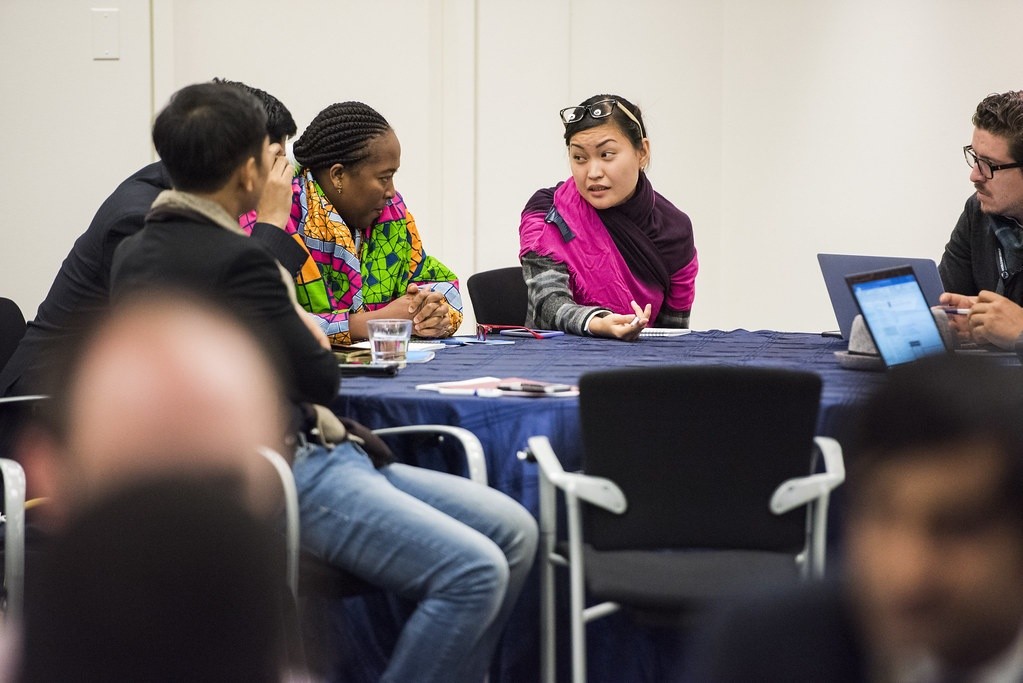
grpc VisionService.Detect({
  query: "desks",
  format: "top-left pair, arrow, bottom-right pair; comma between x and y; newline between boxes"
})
327,331 -> 1023,683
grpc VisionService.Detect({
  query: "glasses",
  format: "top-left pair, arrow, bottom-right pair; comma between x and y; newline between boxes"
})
559,98 -> 644,139
963,144 -> 1023,180
475,322 -> 544,341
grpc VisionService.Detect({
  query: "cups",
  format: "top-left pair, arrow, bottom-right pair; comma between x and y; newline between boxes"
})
367,320 -> 413,368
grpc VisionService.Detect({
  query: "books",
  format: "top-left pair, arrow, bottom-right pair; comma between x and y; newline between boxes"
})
347,335 -> 515,364
415,376 -> 579,398
639,327 -> 692,337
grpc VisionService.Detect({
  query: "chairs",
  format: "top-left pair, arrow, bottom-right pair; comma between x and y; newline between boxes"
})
0,266 -> 850,683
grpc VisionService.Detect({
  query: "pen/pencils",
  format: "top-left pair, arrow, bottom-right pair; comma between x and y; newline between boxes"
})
410,341 -> 469,346
942,308 -> 971,315
437,387 -> 504,397
630,316 -> 640,328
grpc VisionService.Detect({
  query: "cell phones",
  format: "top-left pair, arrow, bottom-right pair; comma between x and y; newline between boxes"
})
500,329 -> 564,338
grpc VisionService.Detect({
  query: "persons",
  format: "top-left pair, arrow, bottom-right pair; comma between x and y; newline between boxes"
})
937,90 -> 1023,351
0,77 -> 539,683
828,355 -> 1023,683
518,95 -> 699,341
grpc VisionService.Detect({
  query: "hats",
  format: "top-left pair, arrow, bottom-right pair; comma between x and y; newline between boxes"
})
833,308 -> 953,368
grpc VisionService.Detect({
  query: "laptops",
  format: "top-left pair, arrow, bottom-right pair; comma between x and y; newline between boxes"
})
817,253 -> 1018,372
